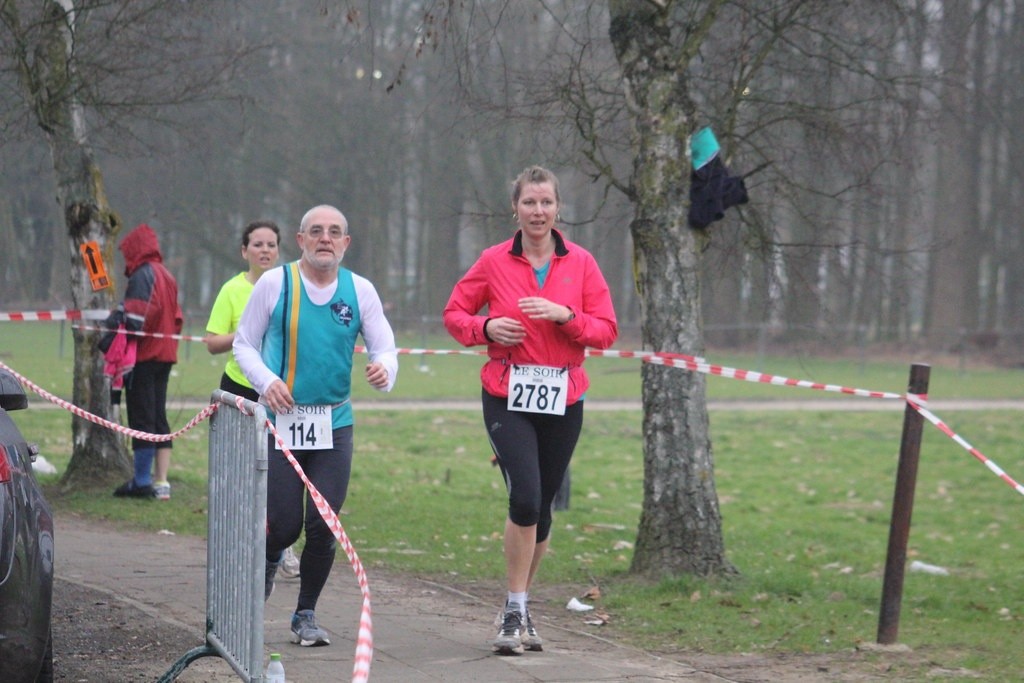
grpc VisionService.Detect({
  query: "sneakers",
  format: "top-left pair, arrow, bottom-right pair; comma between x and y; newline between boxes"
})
289,609 -> 330,647
265,550 -> 284,602
278,546 -> 301,578
493,599 -> 543,650
492,598 -> 524,653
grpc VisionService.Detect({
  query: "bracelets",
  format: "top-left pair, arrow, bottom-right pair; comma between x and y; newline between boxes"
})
570,308 -> 574,320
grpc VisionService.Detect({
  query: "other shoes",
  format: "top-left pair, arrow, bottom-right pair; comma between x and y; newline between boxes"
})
152,481 -> 171,500
113,477 -> 154,499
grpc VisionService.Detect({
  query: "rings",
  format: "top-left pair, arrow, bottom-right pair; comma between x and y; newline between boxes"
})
538,308 -> 542,313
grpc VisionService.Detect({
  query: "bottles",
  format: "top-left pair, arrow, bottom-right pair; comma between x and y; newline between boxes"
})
266,654 -> 285,683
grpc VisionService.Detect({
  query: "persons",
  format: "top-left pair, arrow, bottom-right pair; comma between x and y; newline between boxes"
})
207,219 -> 302,580
232,204 -> 399,646
442,167 -> 618,657
111,224 -> 182,500
